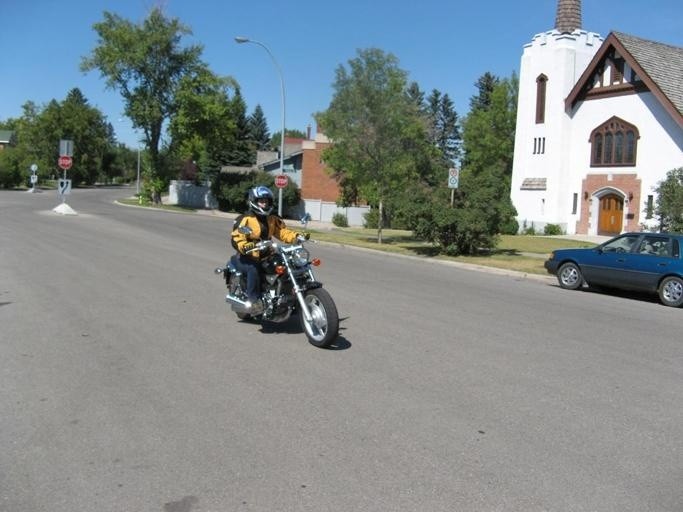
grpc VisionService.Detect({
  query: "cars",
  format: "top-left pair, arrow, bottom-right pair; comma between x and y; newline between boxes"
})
544,231 -> 683,307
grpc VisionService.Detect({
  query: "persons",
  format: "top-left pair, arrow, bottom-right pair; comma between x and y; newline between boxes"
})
229,185 -> 311,318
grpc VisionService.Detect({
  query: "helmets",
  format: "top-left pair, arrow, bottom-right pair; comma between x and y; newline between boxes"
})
248,186 -> 273,216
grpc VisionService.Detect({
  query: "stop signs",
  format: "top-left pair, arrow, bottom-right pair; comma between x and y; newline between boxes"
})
275,175 -> 287,189
59,155 -> 72,170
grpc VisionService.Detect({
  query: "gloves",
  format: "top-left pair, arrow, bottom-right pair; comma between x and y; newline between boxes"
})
300,232 -> 310,240
243,242 -> 254,250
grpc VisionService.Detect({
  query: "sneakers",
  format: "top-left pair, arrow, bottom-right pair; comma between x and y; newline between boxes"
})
250,302 -> 263,317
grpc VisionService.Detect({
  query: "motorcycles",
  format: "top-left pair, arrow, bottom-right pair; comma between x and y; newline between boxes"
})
215,213 -> 339,348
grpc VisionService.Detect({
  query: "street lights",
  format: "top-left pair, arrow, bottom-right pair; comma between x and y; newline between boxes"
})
119,119 -> 141,193
236,36 -> 286,216
